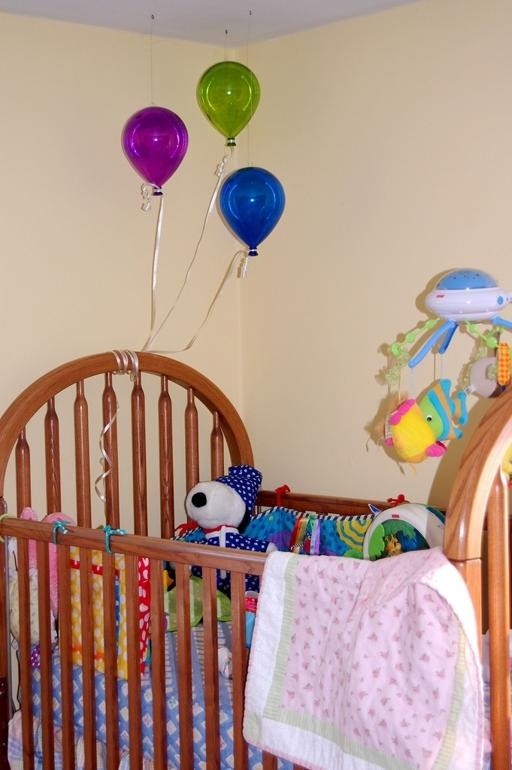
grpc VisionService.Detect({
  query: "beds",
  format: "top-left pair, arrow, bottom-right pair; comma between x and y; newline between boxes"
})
0,347 -> 511,768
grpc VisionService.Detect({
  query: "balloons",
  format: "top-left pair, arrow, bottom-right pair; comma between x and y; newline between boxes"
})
197,61 -> 262,147
120,105 -> 192,198
219,166 -> 286,257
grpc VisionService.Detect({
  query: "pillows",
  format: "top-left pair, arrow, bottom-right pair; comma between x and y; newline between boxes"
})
163,568 -> 231,631
